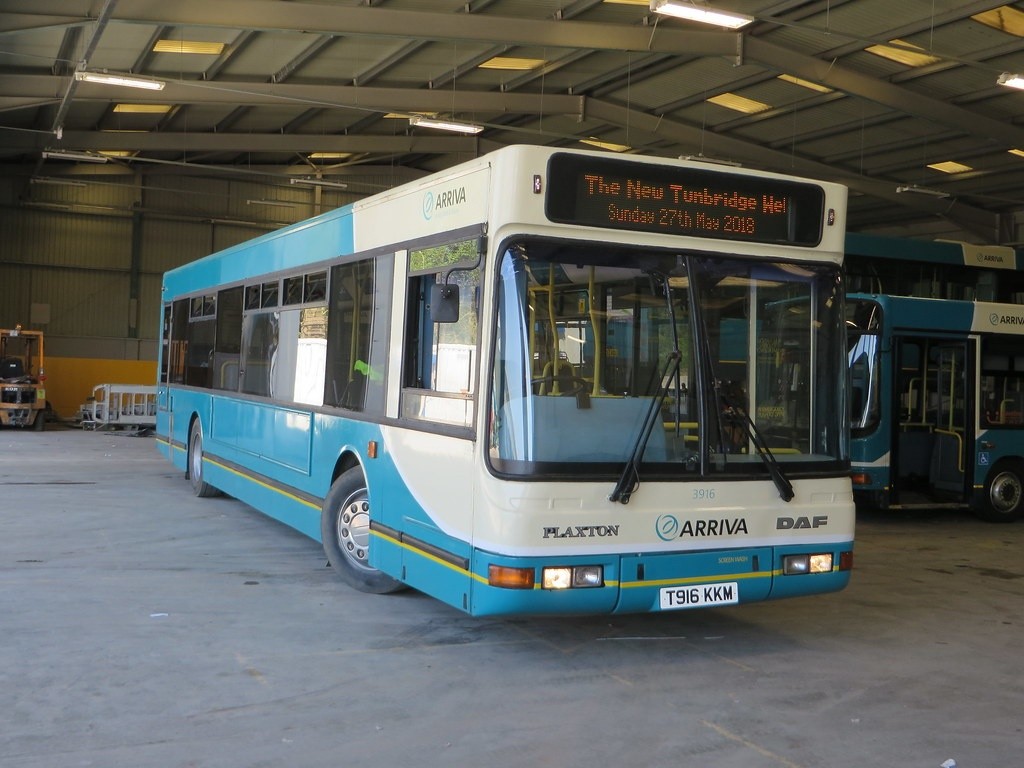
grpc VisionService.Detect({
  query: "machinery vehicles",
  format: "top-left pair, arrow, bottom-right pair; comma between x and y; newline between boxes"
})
0,323 -> 46,431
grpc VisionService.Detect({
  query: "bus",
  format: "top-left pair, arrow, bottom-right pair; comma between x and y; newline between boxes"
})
762,231 -> 1024,524
154,143 -> 855,617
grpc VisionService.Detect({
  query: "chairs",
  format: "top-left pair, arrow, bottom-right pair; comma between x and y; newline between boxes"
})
538,360 -> 577,396
987,399 -> 1024,424
